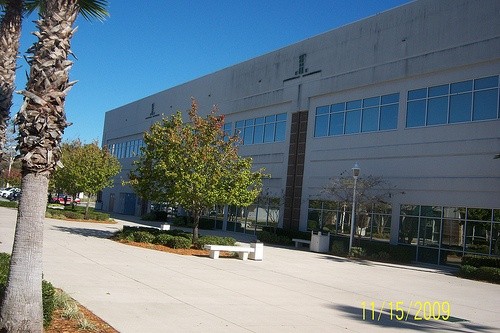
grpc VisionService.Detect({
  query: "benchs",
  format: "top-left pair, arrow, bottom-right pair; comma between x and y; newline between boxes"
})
292,239 -> 311,248
204,244 -> 255,260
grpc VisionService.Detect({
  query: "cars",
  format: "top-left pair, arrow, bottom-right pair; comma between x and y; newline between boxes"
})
0,186 -> 21,201
51,194 -> 80,205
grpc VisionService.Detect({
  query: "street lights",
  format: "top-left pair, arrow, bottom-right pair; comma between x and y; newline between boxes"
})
347,163 -> 361,258
6,146 -> 17,187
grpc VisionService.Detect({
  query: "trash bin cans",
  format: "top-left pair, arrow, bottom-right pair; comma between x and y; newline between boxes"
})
309,228 -> 333,254
